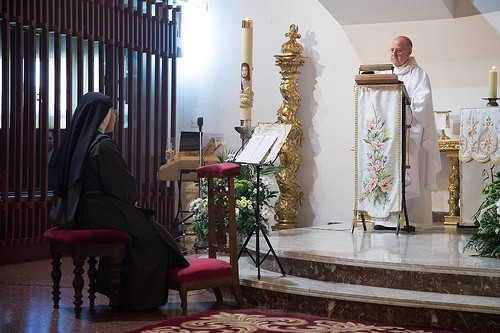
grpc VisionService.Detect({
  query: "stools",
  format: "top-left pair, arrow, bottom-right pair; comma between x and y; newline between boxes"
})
44,227 -> 131,320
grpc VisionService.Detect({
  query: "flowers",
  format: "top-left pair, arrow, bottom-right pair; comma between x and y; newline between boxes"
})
189,148 -> 280,244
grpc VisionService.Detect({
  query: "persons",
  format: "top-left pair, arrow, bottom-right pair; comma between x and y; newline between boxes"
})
361,35 -> 441,231
49,94 -> 189,312
240,63 -> 251,90
37,129 -> 55,180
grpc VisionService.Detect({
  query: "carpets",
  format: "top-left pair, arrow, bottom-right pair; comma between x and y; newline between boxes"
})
127,308 -> 448,333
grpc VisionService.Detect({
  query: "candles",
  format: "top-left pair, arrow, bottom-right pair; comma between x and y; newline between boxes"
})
489,65 -> 498,98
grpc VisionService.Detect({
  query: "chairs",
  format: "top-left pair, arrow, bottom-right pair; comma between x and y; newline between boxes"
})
158,161 -> 243,314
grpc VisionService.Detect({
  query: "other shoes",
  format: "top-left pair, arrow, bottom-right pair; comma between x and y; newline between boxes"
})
375,224 -> 397,230
404,225 -> 415,232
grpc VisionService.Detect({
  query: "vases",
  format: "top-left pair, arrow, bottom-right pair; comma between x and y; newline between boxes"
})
223,232 -> 244,246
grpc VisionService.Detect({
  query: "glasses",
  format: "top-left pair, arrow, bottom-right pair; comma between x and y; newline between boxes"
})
113,108 -> 117,114
388,48 -> 403,53
